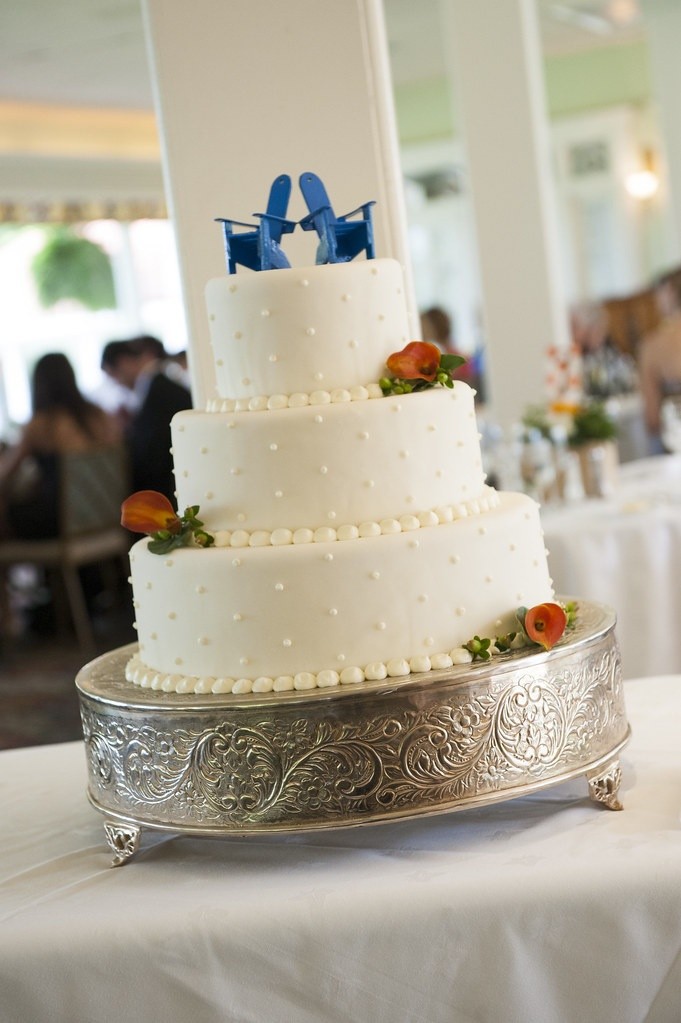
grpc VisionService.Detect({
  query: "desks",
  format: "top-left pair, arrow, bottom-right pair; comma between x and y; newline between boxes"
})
0,673 -> 681,1023
539,452 -> 681,682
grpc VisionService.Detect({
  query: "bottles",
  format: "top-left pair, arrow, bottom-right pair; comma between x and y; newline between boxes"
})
486,424 -> 583,509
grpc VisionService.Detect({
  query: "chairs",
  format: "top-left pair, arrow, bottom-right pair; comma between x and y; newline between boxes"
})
1,440 -> 128,651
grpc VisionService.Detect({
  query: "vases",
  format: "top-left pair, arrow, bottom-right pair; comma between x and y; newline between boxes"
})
577,441 -> 620,501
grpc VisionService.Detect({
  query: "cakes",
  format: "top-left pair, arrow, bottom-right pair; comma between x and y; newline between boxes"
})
119,170 -> 578,696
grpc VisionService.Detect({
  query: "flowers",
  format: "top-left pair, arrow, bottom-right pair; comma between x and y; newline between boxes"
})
120,489 -> 215,556
378,342 -> 465,398
541,317 -> 638,447
464,601 -> 581,662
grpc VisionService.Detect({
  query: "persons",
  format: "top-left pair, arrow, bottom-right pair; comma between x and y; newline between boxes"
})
420,308 -> 479,405
0,335 -> 193,654
